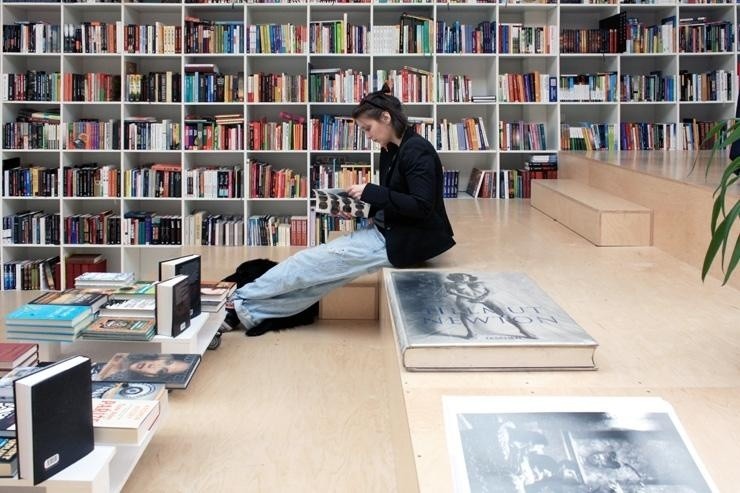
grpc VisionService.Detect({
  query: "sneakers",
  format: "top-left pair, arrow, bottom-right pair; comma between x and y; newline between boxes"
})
223,308 -> 240,332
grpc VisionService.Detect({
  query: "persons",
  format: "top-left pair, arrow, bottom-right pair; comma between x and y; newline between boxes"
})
224,82 -> 457,326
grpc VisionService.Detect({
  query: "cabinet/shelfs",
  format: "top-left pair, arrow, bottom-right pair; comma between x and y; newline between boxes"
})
562,0 -> 736,151
371,2 -> 559,199
2,2 -> 181,292
181,0 -> 371,250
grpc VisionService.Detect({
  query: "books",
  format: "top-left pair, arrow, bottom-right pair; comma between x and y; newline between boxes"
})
386,269 -> 599,372
0,253 -> 238,487
3,21 -> 733,245
